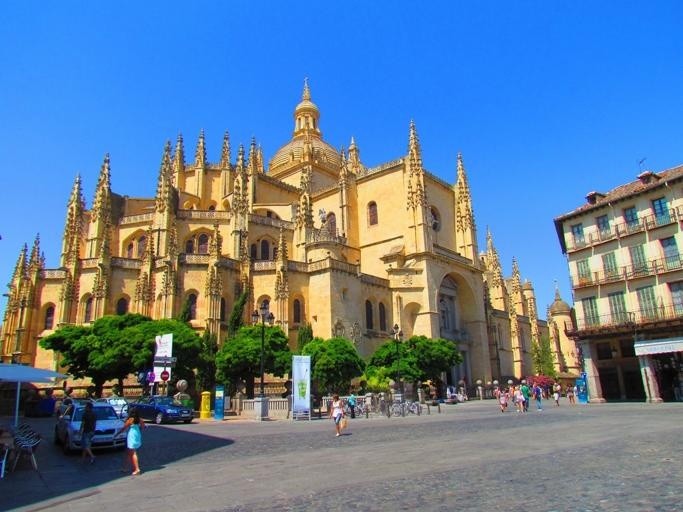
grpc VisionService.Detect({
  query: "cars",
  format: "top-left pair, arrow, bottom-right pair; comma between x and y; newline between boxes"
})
344,397 -> 423,416
57,396 -> 130,418
56,403 -> 128,456
129,395 -> 193,425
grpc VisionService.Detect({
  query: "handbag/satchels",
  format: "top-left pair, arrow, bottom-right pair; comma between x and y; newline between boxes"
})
341,419 -> 348,428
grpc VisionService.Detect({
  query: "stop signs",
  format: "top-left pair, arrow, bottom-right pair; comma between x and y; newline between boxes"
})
161,371 -> 170,381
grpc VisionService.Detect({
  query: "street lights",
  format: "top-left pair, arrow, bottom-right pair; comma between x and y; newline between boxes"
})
390,323 -> 404,416
252,303 -> 275,421
1,292 -> 31,364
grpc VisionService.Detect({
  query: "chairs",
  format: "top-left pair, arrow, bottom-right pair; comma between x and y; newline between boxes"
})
11,420 -> 43,473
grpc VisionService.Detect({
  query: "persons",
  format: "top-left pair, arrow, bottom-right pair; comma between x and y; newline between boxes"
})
113,408 -> 144,475
329,395 -> 344,437
346,391 -> 356,418
78,403 -> 97,466
494,381 -> 577,414
672,376 -> 681,401
446,384 -> 465,403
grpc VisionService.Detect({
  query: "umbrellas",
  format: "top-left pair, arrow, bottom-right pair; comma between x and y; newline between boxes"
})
0,363 -> 72,427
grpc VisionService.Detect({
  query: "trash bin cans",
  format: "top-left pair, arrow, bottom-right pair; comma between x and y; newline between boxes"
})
181,399 -> 194,409
366,393 -> 375,412
40,399 -> 57,416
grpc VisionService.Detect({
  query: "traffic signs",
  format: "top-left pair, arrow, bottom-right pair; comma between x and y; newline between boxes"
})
153,363 -> 176,368
154,356 -> 177,362
